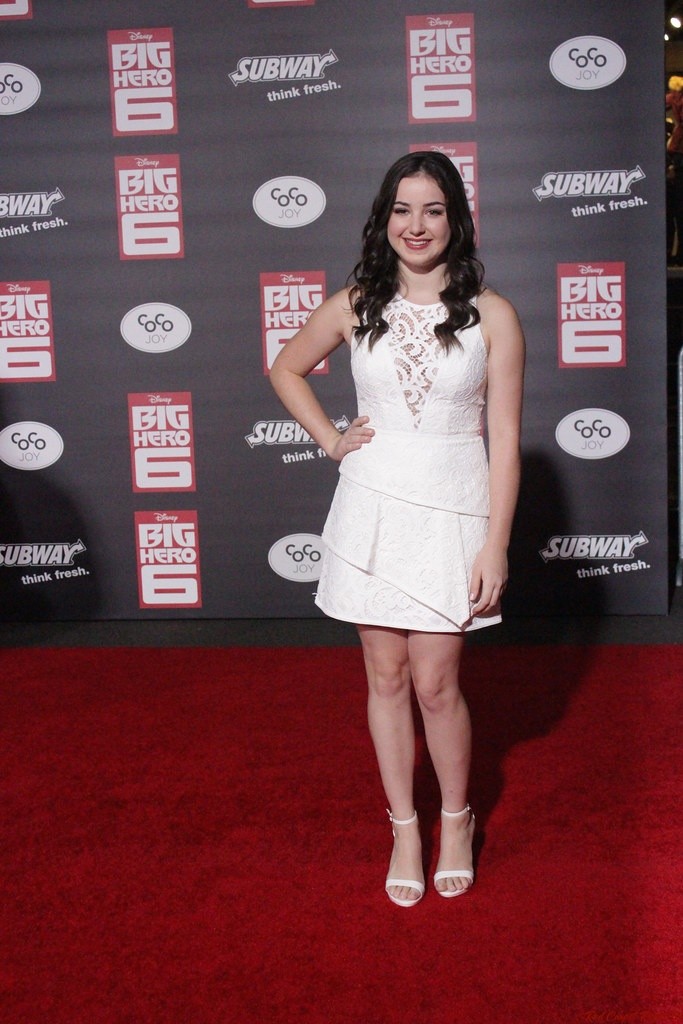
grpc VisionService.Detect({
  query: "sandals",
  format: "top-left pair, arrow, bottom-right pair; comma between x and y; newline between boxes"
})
386,808 -> 425,907
433,804 -> 476,898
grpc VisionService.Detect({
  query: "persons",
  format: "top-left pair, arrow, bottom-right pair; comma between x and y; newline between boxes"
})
270,150 -> 525,907
